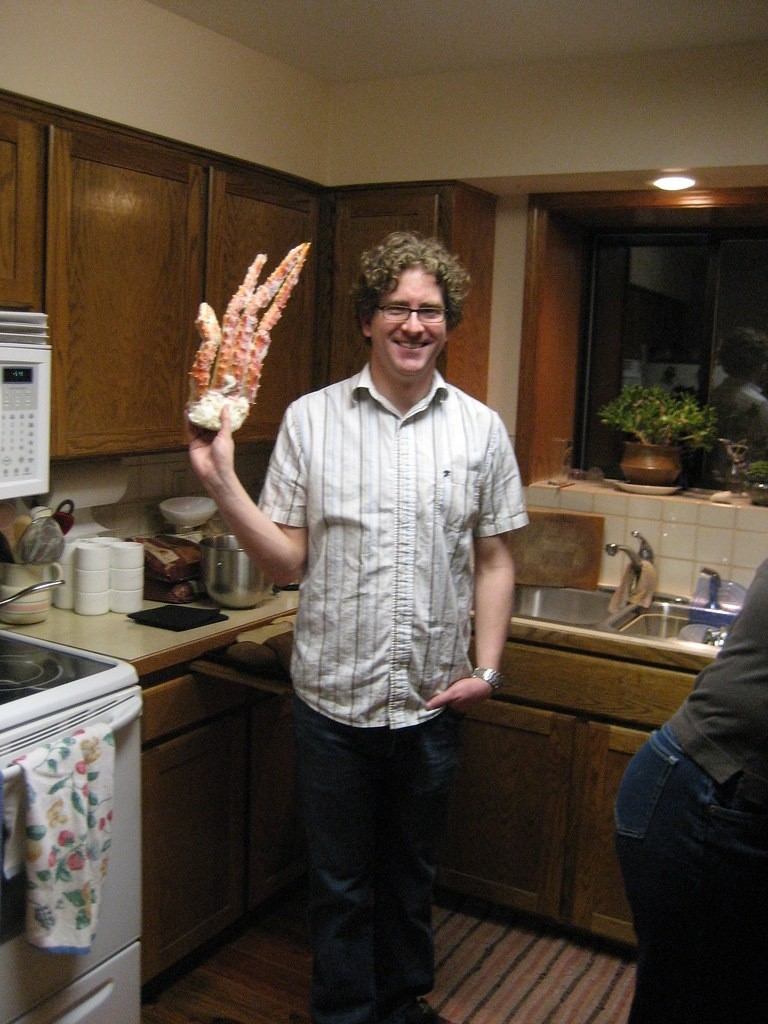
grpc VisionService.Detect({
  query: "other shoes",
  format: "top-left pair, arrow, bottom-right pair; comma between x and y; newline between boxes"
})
383,998 -> 454,1024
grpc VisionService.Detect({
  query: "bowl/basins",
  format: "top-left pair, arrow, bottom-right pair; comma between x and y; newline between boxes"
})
159,497 -> 216,525
0,585 -> 51,625
54,538 -> 144,615
199,535 -> 273,609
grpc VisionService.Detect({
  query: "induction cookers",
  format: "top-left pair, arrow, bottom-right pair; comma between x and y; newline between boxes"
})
0,629 -> 139,732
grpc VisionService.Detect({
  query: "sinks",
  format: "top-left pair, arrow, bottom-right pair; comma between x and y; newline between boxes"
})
609,605 -> 690,640
511,587 -> 615,624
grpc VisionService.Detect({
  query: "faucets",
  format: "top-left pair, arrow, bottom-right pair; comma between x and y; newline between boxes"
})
606,530 -> 653,596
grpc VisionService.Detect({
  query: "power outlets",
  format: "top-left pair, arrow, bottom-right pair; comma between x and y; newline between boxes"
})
171,471 -> 188,497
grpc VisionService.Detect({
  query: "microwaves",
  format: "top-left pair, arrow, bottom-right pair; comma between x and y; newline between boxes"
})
0,342 -> 53,500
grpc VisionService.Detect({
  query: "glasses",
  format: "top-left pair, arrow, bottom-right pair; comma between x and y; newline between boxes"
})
374,304 -> 450,324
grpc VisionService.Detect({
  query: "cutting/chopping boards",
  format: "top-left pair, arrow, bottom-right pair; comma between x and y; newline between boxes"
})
497,506 -> 606,589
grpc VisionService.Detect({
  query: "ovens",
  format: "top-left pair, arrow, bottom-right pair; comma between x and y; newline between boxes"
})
0,686 -> 140,1024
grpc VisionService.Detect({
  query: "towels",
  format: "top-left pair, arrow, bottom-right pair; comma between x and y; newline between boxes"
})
2,723 -> 116,956
605,561 -> 655,615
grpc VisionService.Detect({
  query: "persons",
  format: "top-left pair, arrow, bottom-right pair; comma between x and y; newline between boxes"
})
614,559 -> 768,1024
183,231 -> 530,1024
706,327 -> 768,460
659,365 -> 697,401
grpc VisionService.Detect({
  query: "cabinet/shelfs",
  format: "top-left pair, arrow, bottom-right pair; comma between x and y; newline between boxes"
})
433,698 -> 650,946
141,665 -> 302,986
0,88 -> 498,464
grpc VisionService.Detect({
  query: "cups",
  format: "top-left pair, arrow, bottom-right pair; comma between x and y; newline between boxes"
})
0,563 -> 62,603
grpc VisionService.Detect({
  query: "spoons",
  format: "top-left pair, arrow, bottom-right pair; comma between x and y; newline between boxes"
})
0,502 -> 23,563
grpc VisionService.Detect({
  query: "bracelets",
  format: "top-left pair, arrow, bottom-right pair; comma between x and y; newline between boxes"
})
472,666 -> 503,688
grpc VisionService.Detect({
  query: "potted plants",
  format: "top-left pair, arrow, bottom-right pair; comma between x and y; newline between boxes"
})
597,383 -> 718,487
